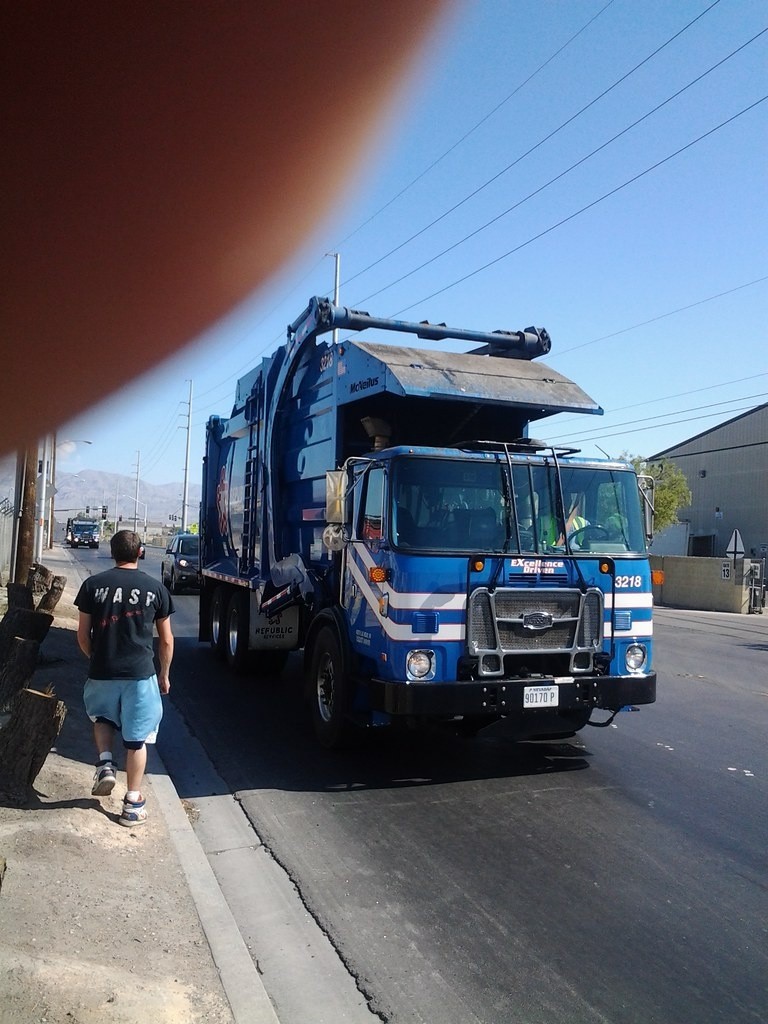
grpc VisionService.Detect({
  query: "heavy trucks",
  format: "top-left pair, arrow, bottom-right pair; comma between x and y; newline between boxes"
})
191,293 -> 667,751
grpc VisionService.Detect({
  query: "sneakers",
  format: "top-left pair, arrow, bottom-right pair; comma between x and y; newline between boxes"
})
91,760 -> 118,796
119,796 -> 148,826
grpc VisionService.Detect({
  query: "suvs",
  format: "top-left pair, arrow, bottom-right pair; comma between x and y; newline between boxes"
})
160,532 -> 199,595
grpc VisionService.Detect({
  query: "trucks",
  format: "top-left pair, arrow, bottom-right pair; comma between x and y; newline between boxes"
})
64,516 -> 101,549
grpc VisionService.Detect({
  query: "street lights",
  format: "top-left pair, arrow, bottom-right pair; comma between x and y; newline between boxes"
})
123,494 -> 148,545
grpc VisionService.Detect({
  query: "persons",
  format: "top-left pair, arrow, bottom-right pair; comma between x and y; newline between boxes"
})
537,487 -> 591,549
73,530 -> 176,826
